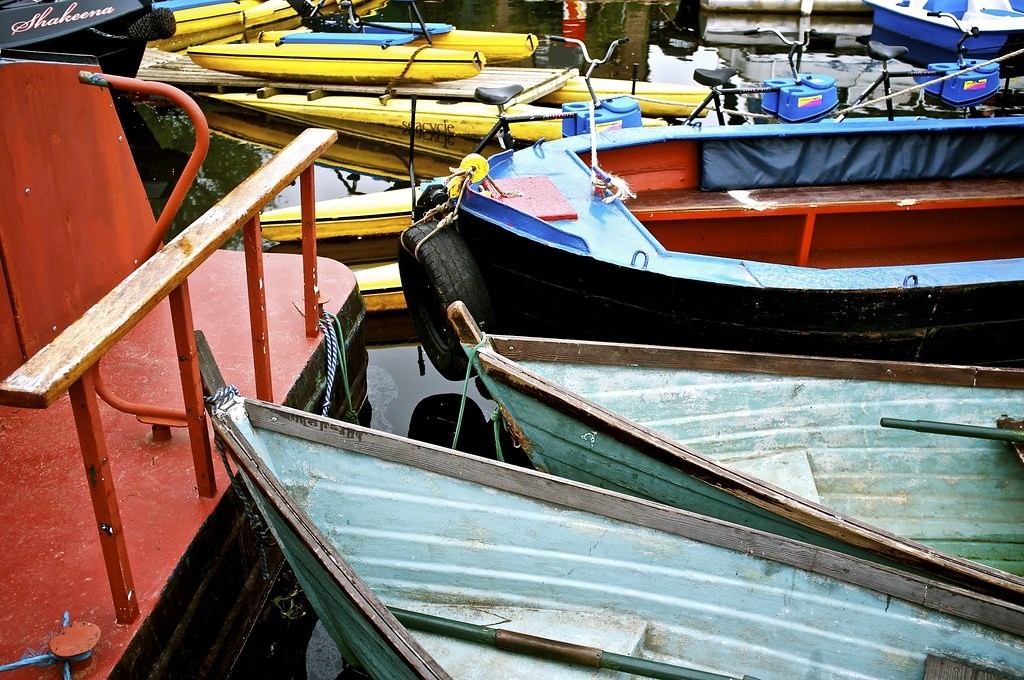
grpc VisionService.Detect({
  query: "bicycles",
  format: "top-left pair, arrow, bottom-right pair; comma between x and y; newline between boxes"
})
684,26 -> 820,125
411,36 -> 625,234
834,9 -> 978,122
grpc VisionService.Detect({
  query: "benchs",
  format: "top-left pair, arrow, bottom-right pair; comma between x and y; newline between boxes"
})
621,175 -> 1024,268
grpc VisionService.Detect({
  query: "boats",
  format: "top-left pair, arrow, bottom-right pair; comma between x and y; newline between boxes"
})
149,0 -> 717,145
440,114 -> 1023,347
866,0 -> 1024,53
445,301 -> 1023,598
207,394 -> 1023,680
